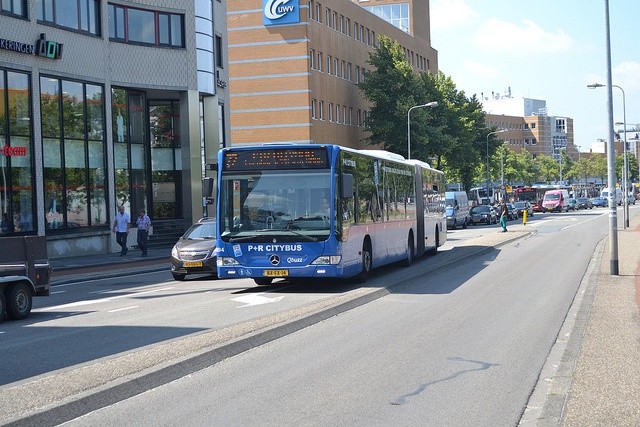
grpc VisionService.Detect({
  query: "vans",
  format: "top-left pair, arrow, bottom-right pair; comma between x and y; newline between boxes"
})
445,191 -> 470,230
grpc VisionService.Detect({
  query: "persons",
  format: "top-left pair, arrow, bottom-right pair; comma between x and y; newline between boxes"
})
135,209 -> 152,257
499,199 -> 508,232
314,198 -> 337,224
112,206 -> 131,256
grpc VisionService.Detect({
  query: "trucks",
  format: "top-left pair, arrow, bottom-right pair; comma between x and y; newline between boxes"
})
599,188 -> 622,206
542,190 -> 569,213
0,235 -> 50,322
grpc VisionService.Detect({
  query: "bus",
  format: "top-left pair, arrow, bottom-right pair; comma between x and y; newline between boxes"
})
468,188 -> 494,210
516,188 -> 556,212
216,143 -> 447,285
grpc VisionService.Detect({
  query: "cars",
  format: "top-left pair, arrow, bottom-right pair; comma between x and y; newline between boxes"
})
514,202 -> 528,217
496,204 -> 513,220
170,217 -> 217,280
527,202 -> 533,217
469,205 -> 497,226
590,197 -> 608,208
511,204 -> 518,220
628,192 -> 635,205
569,198 -> 579,211
578,198 -> 592,209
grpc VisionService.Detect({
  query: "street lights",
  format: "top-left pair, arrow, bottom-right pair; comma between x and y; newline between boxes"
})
487,129 -> 508,205
588,84 -> 628,228
408,101 -> 439,160
501,141 -> 510,187
615,122 -> 640,133
